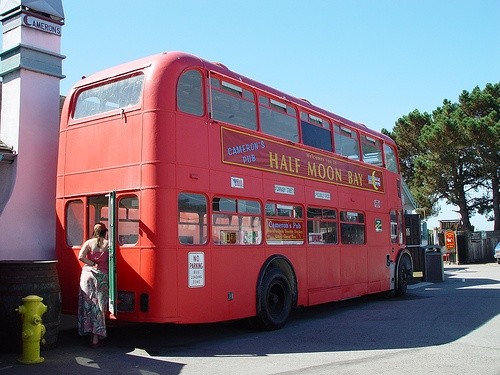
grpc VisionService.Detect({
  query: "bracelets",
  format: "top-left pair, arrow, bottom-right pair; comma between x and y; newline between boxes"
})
93,262 -> 95,265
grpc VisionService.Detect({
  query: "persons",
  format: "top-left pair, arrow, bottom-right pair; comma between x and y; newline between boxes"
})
77,223 -> 109,348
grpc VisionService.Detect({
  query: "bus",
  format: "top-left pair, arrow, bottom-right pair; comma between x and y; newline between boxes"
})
55,50 -> 412,331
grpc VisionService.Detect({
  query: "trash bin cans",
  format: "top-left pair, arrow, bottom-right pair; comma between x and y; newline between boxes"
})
424,245 -> 444,283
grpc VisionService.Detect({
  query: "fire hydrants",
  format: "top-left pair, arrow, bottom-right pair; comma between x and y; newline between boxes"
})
18,293 -> 47,366
